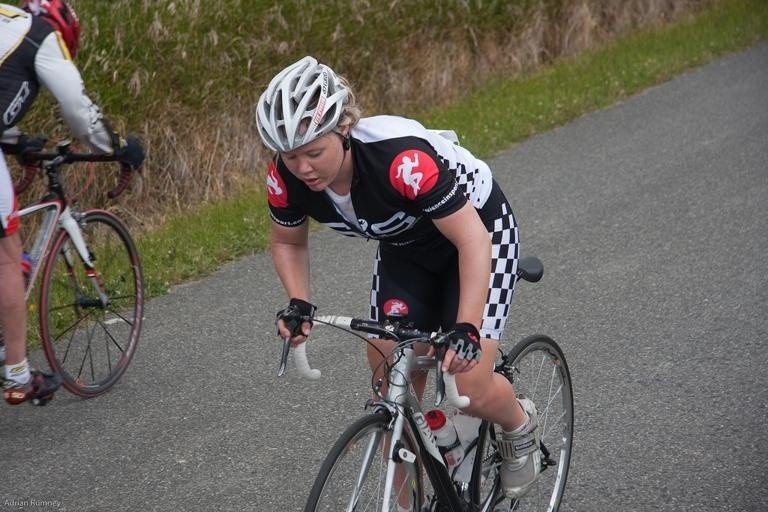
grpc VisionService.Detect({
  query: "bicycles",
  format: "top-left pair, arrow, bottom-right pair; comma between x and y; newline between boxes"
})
273,259 -> 576,512
0,131 -> 148,407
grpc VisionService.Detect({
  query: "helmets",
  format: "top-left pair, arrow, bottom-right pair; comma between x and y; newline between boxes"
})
19,0 -> 84,64
254,55 -> 349,155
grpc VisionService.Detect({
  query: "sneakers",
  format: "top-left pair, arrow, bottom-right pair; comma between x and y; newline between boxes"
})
396,489 -> 428,512
495,393 -> 542,497
1,372 -> 63,404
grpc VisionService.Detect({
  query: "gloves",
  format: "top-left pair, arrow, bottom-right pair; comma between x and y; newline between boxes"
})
108,130 -> 147,169
14,128 -> 41,169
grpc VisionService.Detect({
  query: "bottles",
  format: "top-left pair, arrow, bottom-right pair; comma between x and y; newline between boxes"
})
425,409 -> 467,466
453,396 -> 482,484
21,248 -> 30,288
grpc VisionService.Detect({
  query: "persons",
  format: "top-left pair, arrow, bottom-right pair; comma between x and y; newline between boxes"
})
0,0 -> 144,405
254,53 -> 542,511
